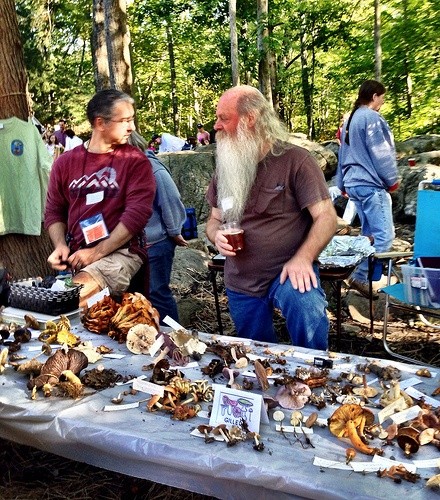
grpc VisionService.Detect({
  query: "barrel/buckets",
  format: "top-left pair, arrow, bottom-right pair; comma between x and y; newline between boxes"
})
400,265 -> 440,308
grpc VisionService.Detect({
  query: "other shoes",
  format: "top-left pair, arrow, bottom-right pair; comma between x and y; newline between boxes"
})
348,274 -> 398,299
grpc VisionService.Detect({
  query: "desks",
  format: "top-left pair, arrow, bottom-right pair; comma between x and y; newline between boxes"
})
0,312 -> 440,500
207,236 -> 374,352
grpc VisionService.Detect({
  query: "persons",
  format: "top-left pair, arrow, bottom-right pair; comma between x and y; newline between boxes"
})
337,80 -> 400,299
328,186 -> 358,226
43,119 -> 84,160
148,120 -> 217,154
206,85 -> 337,351
45,90 -> 156,317
336,122 -> 344,144
127,131 -> 187,327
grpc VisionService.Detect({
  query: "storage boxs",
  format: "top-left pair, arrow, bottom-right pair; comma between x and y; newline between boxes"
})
8,276 -> 85,315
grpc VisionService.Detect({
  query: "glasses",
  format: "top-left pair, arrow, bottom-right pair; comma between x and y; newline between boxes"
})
107,117 -> 135,124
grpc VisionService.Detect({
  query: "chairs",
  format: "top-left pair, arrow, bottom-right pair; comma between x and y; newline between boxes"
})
374,179 -> 440,367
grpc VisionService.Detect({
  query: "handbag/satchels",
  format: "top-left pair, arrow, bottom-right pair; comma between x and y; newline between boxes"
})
182,208 -> 199,239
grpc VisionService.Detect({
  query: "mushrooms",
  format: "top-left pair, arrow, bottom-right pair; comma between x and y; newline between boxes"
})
0,291 -> 439,489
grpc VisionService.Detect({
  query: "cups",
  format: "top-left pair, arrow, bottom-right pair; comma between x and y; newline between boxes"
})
218,222 -> 244,250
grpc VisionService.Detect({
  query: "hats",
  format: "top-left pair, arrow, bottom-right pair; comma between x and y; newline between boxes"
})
197,123 -> 203,128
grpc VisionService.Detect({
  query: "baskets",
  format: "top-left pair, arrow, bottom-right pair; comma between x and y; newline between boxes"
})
7,276 -> 85,316
